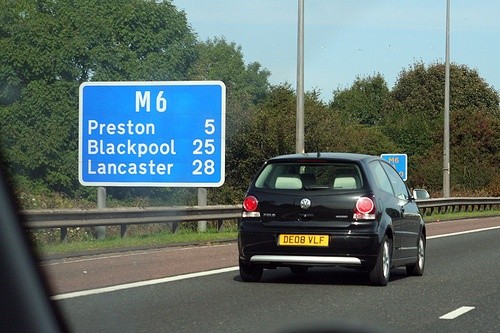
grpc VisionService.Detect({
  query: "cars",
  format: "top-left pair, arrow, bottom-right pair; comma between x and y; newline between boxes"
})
238,153 -> 430,286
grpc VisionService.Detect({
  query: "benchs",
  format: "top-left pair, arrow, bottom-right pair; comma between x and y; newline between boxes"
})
275,175 -> 362,190
